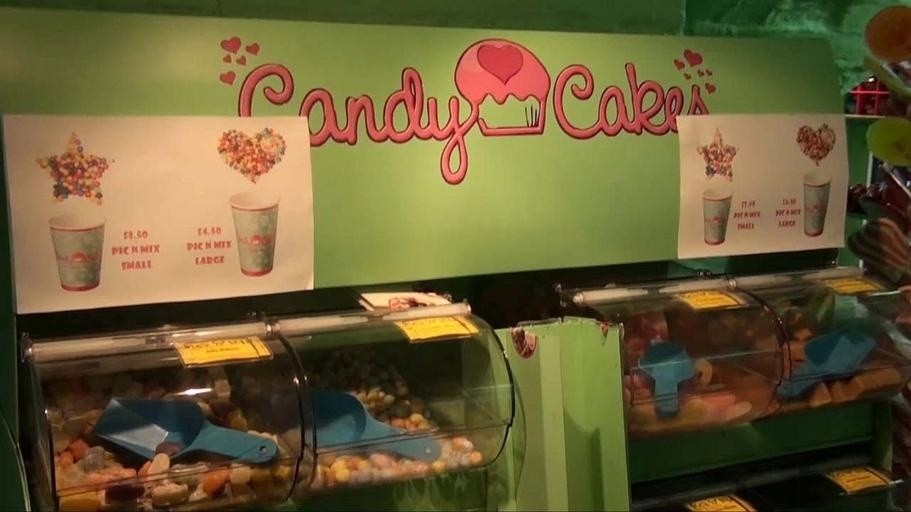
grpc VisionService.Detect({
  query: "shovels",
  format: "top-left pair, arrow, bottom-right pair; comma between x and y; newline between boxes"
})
95,396 -> 277,463
778,324 -> 876,399
288,391 -> 441,461
638,341 -> 696,414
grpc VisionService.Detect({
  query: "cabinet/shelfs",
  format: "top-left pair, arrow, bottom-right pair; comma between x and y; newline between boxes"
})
28,312 -> 509,511
548,269 -> 911,510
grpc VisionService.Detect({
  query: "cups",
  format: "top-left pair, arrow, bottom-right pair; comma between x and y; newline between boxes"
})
49,215 -> 106,291
703,189 -> 733,245
803,173 -> 832,236
231,191 -> 279,276
